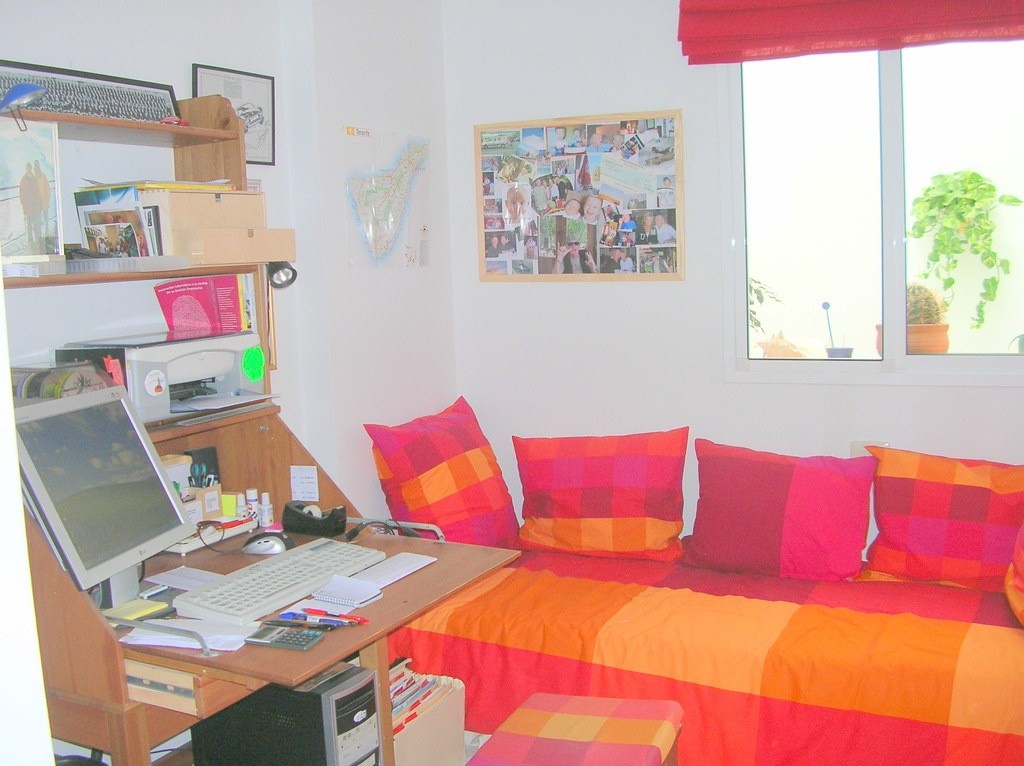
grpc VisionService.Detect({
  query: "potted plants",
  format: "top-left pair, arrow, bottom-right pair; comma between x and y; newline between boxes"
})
907,169 -> 1021,330
876,283 -> 949,358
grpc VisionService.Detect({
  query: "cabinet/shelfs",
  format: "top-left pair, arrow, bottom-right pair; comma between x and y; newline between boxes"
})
0,92 -> 281,442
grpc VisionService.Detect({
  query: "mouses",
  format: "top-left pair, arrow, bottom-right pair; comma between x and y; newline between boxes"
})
241,532 -> 295,553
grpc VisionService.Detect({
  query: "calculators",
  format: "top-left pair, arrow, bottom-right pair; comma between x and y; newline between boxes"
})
243,626 -> 324,651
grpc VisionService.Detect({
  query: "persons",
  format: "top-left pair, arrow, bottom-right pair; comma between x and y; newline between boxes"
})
97,236 -> 131,258
482,120 -> 675,273
105,213 -> 122,223
0,74 -> 174,123
20,160 -> 51,242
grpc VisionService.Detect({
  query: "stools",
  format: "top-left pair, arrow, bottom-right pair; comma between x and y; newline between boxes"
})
464,691 -> 683,766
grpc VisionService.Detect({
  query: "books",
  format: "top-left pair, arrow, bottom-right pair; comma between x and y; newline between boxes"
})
312,574 -> 383,608
154,272 -> 256,340
102,598 -> 168,628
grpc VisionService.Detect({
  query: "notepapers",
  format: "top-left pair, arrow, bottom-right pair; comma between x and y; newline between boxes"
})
104,599 -> 167,620
221,494 -> 237,517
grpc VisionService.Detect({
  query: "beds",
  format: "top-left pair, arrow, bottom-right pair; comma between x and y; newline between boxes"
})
362,395 -> 1024,766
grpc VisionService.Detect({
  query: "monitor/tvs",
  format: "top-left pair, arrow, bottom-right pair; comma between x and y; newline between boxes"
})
14,384 -> 199,628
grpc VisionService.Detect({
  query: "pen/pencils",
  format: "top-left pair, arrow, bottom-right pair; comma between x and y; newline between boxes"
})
263,607 -> 369,630
187,473 -> 218,487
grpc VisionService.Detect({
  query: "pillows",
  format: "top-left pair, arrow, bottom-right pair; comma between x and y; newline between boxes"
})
362,395 -> 520,550
511,426 -> 690,559
865,445 -> 1024,593
681,436 -> 879,582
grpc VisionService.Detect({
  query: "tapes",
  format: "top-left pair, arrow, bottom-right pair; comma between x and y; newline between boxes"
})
303,505 -> 343,518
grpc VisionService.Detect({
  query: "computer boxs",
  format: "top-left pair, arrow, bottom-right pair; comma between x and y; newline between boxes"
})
190,665 -> 383,766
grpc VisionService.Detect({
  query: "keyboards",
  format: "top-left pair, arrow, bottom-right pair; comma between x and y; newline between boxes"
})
172,537 -> 387,624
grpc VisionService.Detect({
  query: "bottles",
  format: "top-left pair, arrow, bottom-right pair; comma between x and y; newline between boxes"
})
245,488 -> 258,516
235,493 -> 247,516
259,492 -> 274,527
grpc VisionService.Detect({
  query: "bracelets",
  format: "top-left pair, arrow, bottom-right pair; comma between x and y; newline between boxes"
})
556,260 -> 561,263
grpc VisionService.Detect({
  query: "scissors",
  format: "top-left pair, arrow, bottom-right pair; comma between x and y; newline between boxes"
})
190,461 -> 209,487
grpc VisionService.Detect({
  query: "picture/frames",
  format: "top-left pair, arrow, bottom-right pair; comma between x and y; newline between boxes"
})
192,62 -> 276,166
0,60 -> 181,125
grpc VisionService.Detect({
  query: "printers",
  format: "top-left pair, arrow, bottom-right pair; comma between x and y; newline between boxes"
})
56,329 -> 279,424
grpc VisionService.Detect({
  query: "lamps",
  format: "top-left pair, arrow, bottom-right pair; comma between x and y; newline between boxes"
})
0,83 -> 48,132
266,261 -> 297,289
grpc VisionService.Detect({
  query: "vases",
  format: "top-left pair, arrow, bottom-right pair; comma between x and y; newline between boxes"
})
826,347 -> 853,358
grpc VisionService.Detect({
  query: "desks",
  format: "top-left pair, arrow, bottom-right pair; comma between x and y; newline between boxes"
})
19,406 -> 522,766
